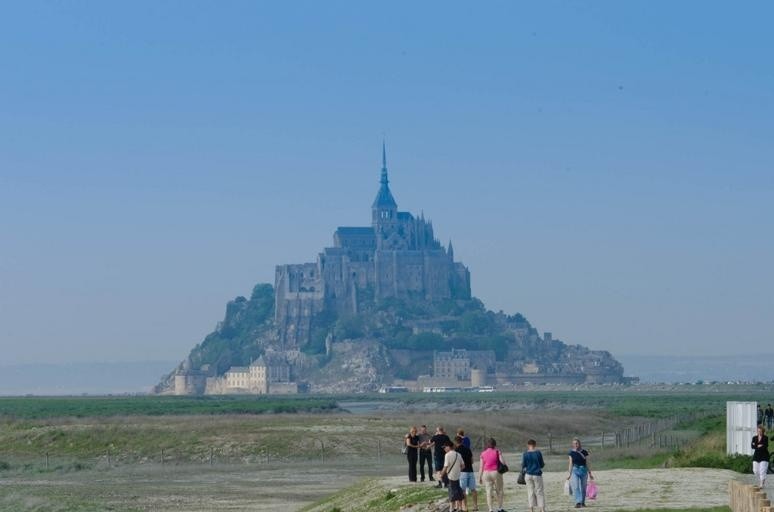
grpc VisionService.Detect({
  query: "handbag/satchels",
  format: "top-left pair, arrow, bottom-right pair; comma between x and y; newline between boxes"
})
564,480 -> 573,496
517,470 -> 526,485
499,464 -> 508,473
586,480 -> 598,499
442,473 -> 449,487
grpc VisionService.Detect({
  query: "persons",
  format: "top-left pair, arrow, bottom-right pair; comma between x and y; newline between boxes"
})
566,437 -> 594,508
479,438 -> 505,512
405,424 -> 479,512
757,404 -> 774,430
751,426 -> 769,488
521,439 -> 545,512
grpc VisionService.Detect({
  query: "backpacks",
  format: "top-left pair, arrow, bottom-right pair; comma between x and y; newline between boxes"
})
400,446 -> 408,454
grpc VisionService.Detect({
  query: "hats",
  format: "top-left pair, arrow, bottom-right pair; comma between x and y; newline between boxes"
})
441,441 -> 454,448
